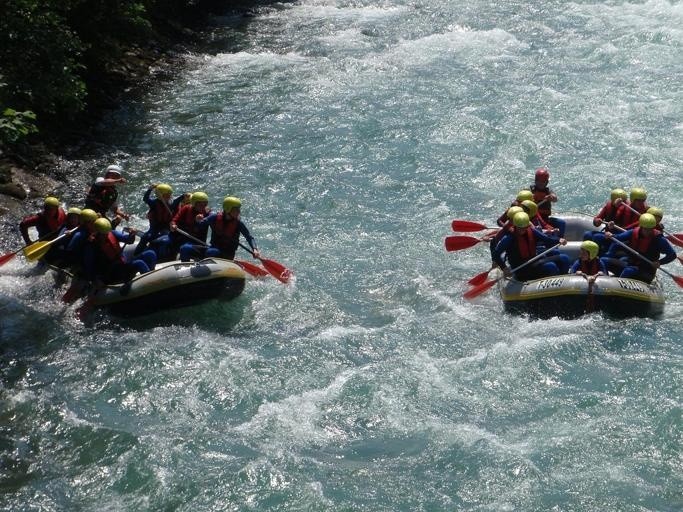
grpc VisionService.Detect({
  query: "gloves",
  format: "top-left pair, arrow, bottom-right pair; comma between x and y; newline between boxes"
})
497,213 -> 665,318
498,213 -> 666,324
91,229 -> 244,318
73,233 -> 246,320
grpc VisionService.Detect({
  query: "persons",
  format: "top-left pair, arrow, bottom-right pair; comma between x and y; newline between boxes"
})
600,213 -> 677,284
488,199 -> 560,235
483,206 -> 560,268
20,197 -> 69,257
172,193 -> 192,213
81,164 -> 130,223
570,240 -> 608,276
64,209 -> 137,278
592,188 -> 651,257
525,167 -> 566,238
133,184 -> 184,258
583,189 -> 626,242
567,207 -> 664,274
56,208 -> 80,247
179,195 -> 260,267
88,218 -> 157,275
156,192 -> 211,258
492,210 -> 570,282
497,190 -> 559,234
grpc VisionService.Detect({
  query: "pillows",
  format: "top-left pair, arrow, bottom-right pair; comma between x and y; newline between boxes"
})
647,206 -> 663,218
611,189 -> 628,203
507,206 -> 523,220
190,192 -> 209,208
580,240 -> 599,260
222,197 -> 241,215
512,212 -> 529,227
44,197 -> 60,208
93,218 -> 112,234
79,209 -> 98,226
183,193 -> 192,204
520,200 -> 538,217
153,183 -> 173,197
105,164 -> 123,176
639,213 -> 656,228
534,168 -> 549,183
516,190 -> 535,203
629,188 -> 647,202
67,208 -> 81,215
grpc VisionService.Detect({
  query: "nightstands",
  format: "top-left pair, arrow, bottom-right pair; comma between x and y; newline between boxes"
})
464,242 -> 561,300
444,228 -> 560,252
451,219 -> 553,233
23,215 -> 101,263
174,226 -> 269,279
609,233 -> 683,287
201,215 -> 294,284
598,219 -> 683,247
0,230 -> 61,268
467,258 -> 513,287
